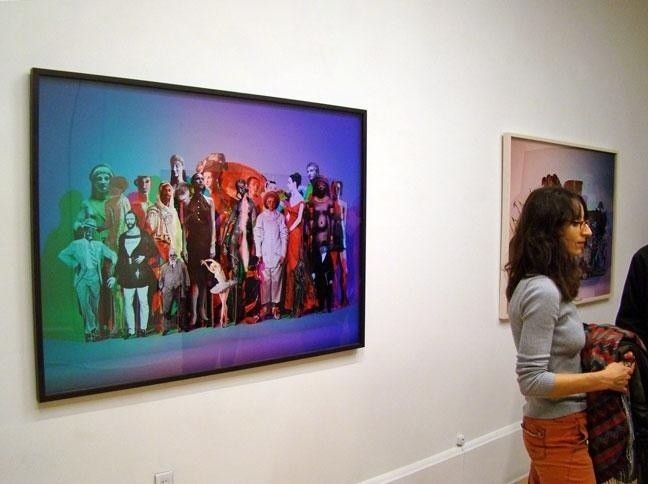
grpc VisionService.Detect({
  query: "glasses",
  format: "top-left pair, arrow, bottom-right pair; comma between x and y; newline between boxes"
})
570,218 -> 591,232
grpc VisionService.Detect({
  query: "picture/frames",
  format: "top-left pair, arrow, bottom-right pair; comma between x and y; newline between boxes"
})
500,132 -> 621,322
30,65 -> 367,404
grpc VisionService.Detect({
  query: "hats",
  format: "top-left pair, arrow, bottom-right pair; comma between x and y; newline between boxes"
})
81,218 -> 99,230
89,164 -> 114,182
263,191 -> 280,209
